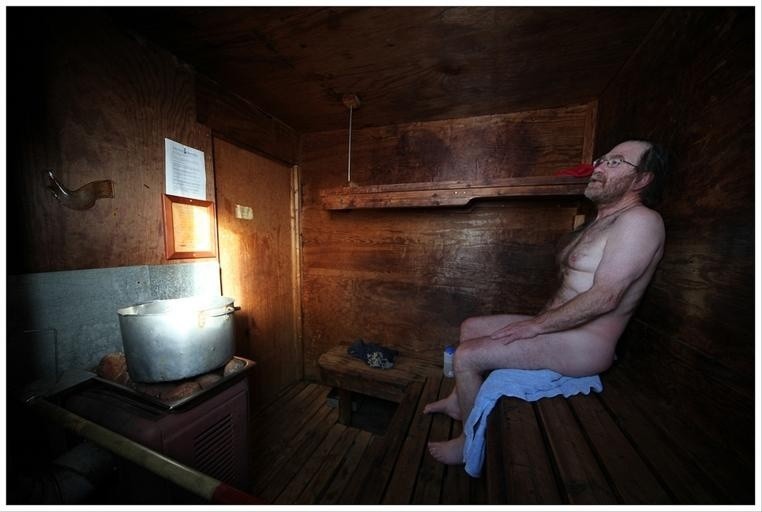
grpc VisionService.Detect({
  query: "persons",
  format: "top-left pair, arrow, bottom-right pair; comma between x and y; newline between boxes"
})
423,138 -> 669,467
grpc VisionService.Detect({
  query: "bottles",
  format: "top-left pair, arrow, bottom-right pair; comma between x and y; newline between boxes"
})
443,347 -> 456,379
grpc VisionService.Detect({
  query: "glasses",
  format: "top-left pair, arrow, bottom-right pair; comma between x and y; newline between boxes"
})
591,157 -> 640,172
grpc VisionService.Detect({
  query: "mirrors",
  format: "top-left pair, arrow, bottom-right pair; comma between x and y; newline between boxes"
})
162,193 -> 218,260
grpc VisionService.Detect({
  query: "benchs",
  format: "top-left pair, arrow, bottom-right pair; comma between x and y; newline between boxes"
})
319,340 -> 437,424
499,348 -> 753,504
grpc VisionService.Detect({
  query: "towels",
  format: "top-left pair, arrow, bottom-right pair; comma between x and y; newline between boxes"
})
463,365 -> 603,477
